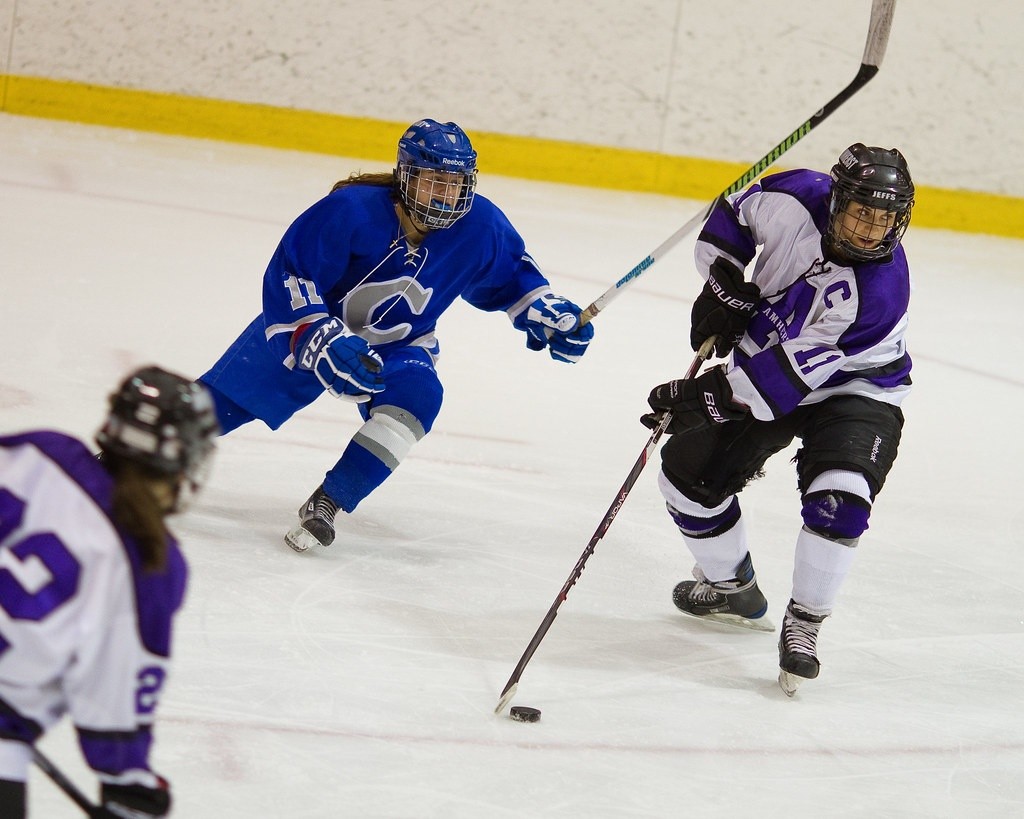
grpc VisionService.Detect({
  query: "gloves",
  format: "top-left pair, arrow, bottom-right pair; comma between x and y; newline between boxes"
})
514,293 -> 594,363
691,256 -> 761,360
639,364 -> 749,437
298,316 -> 387,403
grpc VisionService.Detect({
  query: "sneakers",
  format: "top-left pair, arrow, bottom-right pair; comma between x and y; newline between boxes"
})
672,551 -> 775,632
284,483 -> 342,552
777,597 -> 829,697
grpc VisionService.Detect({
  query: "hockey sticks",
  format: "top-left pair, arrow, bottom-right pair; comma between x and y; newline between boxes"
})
492,333 -> 722,719
30,747 -> 101,819
576,4 -> 897,327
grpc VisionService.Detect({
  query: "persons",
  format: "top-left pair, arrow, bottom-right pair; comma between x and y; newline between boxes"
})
0,364 -> 219,819
94,117 -> 596,554
640,143 -> 916,698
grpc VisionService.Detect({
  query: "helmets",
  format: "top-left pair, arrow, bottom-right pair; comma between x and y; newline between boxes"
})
397,119 -> 477,230
826,142 -> 915,265
96,367 -> 217,513
